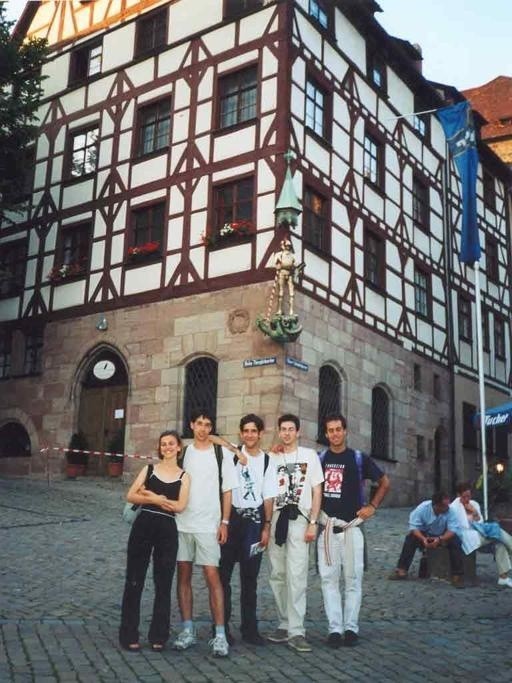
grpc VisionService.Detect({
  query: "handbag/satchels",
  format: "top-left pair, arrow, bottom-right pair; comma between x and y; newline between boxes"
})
121,501 -> 141,526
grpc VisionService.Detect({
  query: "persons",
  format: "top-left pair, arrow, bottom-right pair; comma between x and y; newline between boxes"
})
271,410 -> 389,646
448,481 -> 511,587
118,429 -> 192,652
388,489 -> 464,588
206,413 -> 278,648
275,238 -> 299,316
266,414 -> 325,654
173,406 -> 234,656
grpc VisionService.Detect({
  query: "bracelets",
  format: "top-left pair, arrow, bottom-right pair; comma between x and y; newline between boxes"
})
264,519 -> 270,523
227,443 -> 235,450
369,502 -> 379,513
221,517 -> 230,526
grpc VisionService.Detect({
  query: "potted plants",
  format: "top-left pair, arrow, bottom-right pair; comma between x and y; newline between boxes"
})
104,429 -> 124,477
64,430 -> 88,478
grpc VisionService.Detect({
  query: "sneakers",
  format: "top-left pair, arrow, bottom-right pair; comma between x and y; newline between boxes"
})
344,630 -> 359,646
388,570 -> 409,580
242,632 -> 264,645
208,631 -> 235,657
451,575 -> 466,588
172,628 -> 196,649
497,576 -> 512,588
267,628 -> 289,643
288,635 -> 313,652
328,632 -> 342,648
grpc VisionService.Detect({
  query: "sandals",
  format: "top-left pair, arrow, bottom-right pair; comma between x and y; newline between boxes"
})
123,640 -> 140,651
152,641 -> 164,651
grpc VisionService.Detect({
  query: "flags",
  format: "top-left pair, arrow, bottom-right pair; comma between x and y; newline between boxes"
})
435,98 -> 483,265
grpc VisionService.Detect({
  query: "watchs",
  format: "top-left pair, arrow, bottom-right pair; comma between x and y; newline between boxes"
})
308,518 -> 319,526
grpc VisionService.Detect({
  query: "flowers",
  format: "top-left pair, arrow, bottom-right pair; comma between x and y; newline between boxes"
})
199,216 -> 255,250
127,240 -> 164,260
45,256 -> 88,284
